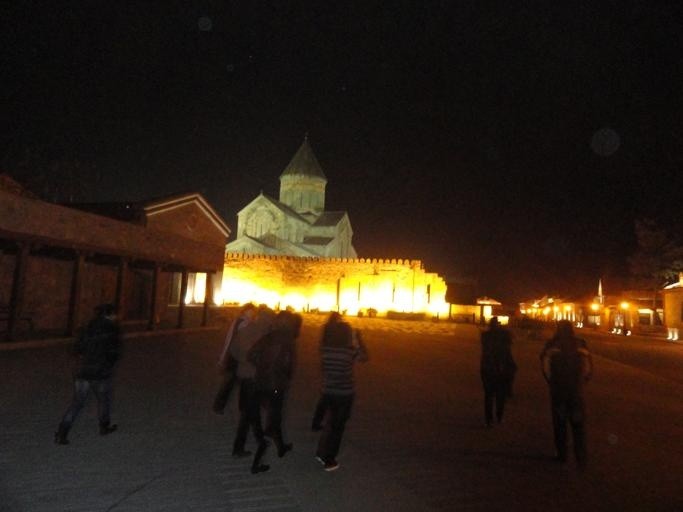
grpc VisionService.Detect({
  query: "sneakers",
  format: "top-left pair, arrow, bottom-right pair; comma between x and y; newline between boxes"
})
324,462 -> 339,472
55,435 -> 69,445
99,423 -> 118,435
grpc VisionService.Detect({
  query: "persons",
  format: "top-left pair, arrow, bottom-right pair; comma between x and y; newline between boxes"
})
480,317 -> 518,428
540,319 -> 593,479
312,312 -> 343,431
315,323 -> 370,471
212,303 -> 303,473
54,302 -> 122,446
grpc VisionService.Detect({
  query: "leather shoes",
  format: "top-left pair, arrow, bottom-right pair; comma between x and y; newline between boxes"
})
231,449 -> 251,458
278,443 -> 292,458
251,465 -> 269,474
